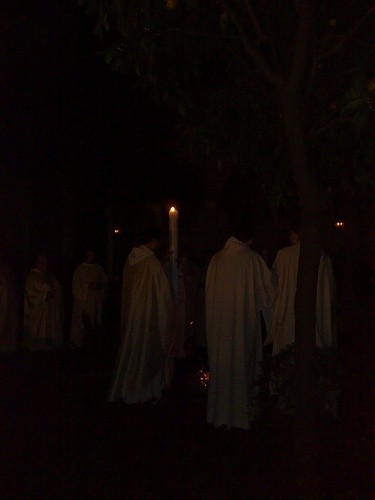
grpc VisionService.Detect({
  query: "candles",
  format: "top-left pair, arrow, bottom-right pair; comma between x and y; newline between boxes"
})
168,207 -> 180,308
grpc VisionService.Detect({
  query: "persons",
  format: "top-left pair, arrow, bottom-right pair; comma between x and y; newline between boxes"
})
204,233 -> 276,430
72,251 -> 107,348
107,234 -> 178,405
261,230 -> 337,415
19,254 -> 63,351
0,263 -> 17,350
